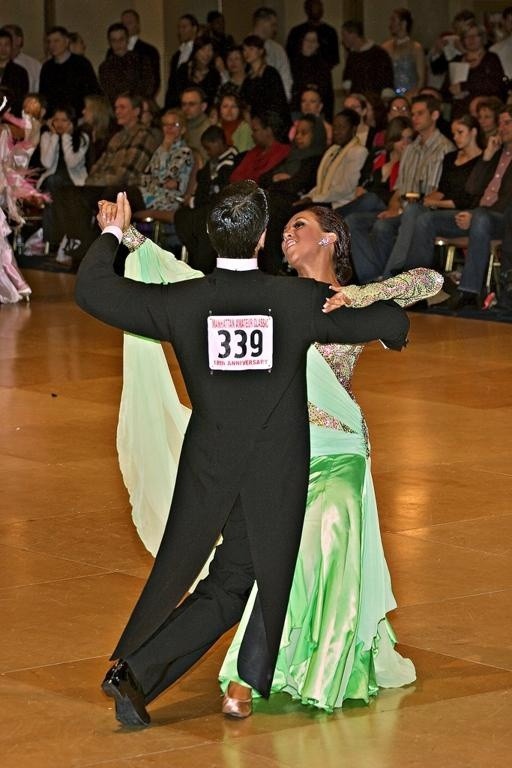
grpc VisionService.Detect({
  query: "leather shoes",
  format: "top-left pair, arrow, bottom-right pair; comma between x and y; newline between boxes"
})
63,235 -> 90,258
427,289 -> 483,317
102,658 -> 151,730
403,299 -> 428,313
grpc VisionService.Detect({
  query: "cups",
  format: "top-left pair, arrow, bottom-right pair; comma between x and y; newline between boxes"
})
405,194 -> 420,206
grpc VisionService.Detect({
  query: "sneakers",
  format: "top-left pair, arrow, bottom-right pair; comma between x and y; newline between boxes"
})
56,233 -> 72,266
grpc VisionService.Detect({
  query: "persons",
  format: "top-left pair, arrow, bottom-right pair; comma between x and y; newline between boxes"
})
441,23 -> 508,127
141,96 -> 161,125
0,24 -> 43,94
165,35 -> 221,109
179,86 -> 213,168
397,107 -> 512,314
92,107 -> 194,274
218,45 -> 249,107
98,23 -> 156,110
213,90 -> 257,151
292,110 -> 368,209
0,28 -> 30,122
285,0 -> 342,125
38,26 -> 101,118
241,33 -> 294,144
451,9 -> 474,33
340,18 -> 396,96
259,113 -> 327,275
476,97 -> 502,133
36,104 -> 90,264
70,179 -> 410,731
163,14 -> 203,108
97,188 -> 444,719
50,94 -> 165,266
22,91 -> 49,122
488,8 -> 512,82
173,126 -> 240,276
288,85 -> 334,147
39,32 -> 87,96
380,7 -> 426,95
106,9 -> 160,67
1,95 -> 53,304
343,94 -> 458,286
206,11 -> 236,63
249,7 -> 294,104
195,106 -> 295,273
381,115 -> 486,279
76,94 -> 124,166
357,115 -> 415,217
343,94 -> 379,151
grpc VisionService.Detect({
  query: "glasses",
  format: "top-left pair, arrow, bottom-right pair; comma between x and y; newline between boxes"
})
350,104 -> 362,109
392,106 -> 408,112
161,121 -> 182,128
181,101 -> 200,106
141,108 -> 153,115
464,34 -> 479,37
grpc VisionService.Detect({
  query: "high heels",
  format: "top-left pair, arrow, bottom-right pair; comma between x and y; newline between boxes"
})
222,678 -> 254,718
16,286 -> 32,302
